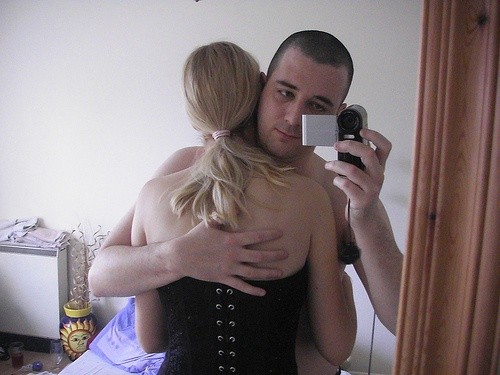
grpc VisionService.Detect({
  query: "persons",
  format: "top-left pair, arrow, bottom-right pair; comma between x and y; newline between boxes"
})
130,41 -> 357,375
88,31 -> 404,375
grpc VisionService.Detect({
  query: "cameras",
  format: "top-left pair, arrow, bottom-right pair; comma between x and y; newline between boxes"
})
302,103 -> 369,177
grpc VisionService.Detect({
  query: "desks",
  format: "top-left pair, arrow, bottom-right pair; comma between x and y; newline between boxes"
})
0,242 -> 70,353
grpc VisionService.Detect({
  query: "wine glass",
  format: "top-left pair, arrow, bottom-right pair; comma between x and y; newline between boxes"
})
50,340 -> 64,374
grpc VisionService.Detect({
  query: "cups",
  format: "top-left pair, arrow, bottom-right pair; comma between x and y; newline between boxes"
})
9,342 -> 24,370
